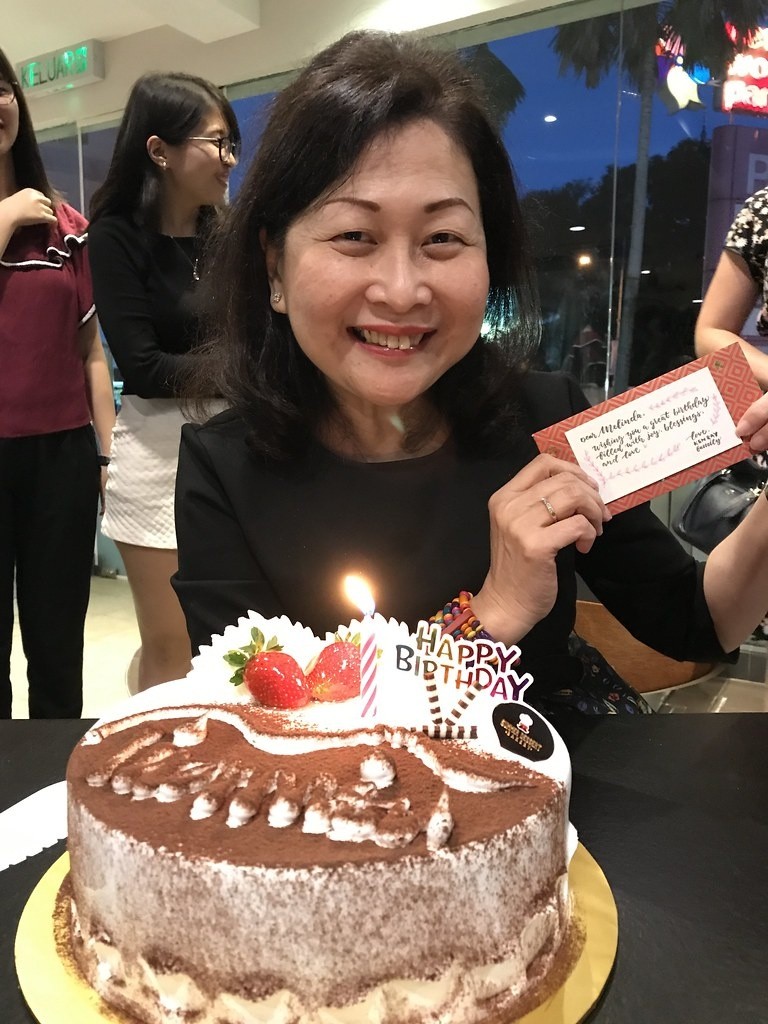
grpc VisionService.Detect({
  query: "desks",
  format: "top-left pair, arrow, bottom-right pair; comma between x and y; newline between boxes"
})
0,711 -> 768,1024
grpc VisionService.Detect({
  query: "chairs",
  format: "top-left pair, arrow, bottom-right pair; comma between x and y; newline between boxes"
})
572,599 -> 728,695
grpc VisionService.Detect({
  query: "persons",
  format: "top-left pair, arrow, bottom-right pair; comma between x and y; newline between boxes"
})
170,31 -> 768,714
694,185 -> 767,496
0,49 -> 113,721
100,71 -> 242,694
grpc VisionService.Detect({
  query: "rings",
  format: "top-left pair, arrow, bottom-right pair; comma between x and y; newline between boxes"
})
540,496 -> 559,522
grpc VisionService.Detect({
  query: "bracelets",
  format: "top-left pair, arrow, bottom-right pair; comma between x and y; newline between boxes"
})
98,452 -> 111,468
421,593 -> 497,647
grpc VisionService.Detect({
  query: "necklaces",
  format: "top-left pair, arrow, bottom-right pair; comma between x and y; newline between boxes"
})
151,219 -> 207,282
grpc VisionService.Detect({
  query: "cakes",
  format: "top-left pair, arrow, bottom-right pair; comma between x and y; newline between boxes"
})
65,631 -> 571,1024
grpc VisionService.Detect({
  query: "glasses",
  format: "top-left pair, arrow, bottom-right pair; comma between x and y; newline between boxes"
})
0,80 -> 19,105
189,137 -> 236,161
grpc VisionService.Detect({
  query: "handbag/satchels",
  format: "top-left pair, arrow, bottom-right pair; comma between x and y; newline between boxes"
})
671,469 -> 763,555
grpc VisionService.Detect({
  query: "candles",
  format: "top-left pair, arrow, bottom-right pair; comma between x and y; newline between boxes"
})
342,575 -> 378,717
